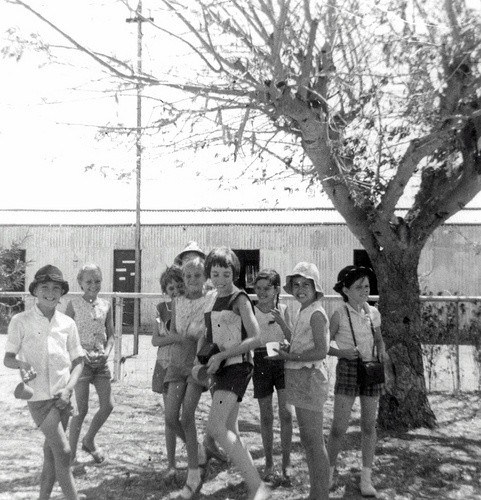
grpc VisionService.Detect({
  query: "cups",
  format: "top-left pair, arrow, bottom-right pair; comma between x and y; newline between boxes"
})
266,342 -> 279,357
13,382 -> 33,400
192,365 -> 211,383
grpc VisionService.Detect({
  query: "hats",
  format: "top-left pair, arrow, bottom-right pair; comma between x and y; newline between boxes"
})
29,265 -> 69,297
333,264 -> 374,293
283,262 -> 324,299
174,241 -> 207,265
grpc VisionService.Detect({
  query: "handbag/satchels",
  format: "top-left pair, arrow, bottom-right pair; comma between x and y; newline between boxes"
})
357,360 -> 385,385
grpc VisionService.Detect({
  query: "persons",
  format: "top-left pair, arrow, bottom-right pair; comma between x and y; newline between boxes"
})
263,261 -> 331,500
4,264 -> 85,500
326,265 -> 388,499
169,260 -> 209,498
64,264 -> 115,463
193,247 -> 270,500
173,241 -> 205,270
251,268 -> 293,483
151,266 -> 206,482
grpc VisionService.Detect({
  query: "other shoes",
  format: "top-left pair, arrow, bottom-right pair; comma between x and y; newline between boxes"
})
328,465 -> 335,490
262,465 -> 276,481
71,456 -> 78,467
175,481 -> 202,500
198,443 -> 207,465
282,465 -> 294,481
82,442 -> 105,463
164,468 -> 177,480
360,466 -> 376,497
254,480 -> 272,500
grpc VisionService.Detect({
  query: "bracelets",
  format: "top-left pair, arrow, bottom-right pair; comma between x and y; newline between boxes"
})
295,353 -> 302,361
